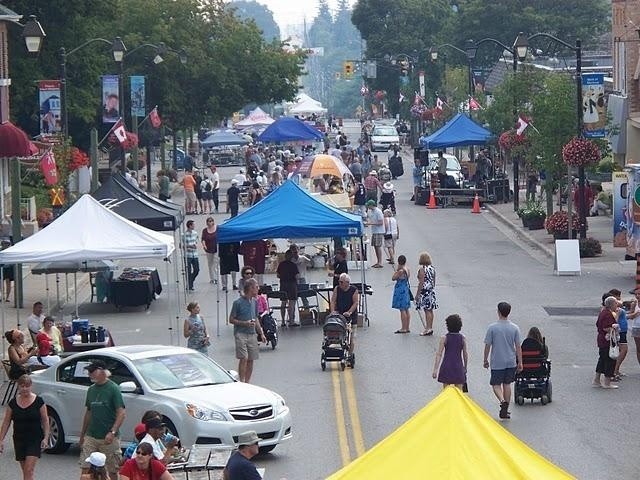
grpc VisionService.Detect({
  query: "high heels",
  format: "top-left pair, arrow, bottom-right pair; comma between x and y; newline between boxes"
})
394,325 -> 434,336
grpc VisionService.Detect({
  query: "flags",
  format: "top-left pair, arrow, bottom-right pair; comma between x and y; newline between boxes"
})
399,93 -> 404,103
436,95 -> 443,110
40,149 -> 58,186
414,94 -> 423,106
515,113 -> 529,137
469,97 -> 479,111
150,108 -> 161,129
113,120 -> 129,149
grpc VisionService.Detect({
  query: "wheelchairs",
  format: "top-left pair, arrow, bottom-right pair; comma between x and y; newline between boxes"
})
515,336 -> 553,405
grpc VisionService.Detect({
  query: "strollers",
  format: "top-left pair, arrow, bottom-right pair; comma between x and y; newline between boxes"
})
321,313 -> 355,371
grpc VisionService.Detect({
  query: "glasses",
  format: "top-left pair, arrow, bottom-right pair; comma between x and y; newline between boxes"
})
135,450 -> 153,456
244,272 -> 252,275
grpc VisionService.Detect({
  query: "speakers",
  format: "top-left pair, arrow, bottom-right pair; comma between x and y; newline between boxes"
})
419,151 -> 429,166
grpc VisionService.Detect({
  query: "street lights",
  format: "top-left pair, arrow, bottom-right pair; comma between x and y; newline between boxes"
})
59,35 -> 128,214
144,48 -> 189,192
118,42 -> 168,179
389,49 -> 423,139
0,14 -> 46,53
428,42 -> 475,163
411,47 -> 447,154
353,57 -> 402,119
464,38 -> 519,211
512,31 -> 586,240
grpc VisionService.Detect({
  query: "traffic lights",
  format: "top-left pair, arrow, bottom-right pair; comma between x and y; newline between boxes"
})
343,62 -> 353,80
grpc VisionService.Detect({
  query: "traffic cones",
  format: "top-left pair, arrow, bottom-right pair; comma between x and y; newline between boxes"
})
427,186 -> 439,209
471,192 -> 482,213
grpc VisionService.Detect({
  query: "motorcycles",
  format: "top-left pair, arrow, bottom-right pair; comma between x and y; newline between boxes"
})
257,305 -> 277,349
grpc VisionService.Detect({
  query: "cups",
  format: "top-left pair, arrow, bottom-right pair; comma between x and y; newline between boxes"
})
165,433 -> 180,445
251,318 -> 256,328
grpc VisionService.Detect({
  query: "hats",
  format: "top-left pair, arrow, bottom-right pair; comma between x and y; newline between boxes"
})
369,170 -> 378,175
365,200 -> 376,208
34,332 -> 52,357
383,182 -> 394,189
85,450 -> 108,469
211,165 -> 216,170
134,423 -> 147,436
231,179 -> 239,184
146,418 -> 167,428
235,430 -> 263,448
83,359 -> 108,373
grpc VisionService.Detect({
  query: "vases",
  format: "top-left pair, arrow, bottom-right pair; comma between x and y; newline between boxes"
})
521,217 -> 528,227
527,217 -> 545,230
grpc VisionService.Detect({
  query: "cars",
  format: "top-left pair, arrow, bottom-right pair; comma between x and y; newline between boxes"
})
209,143 -> 248,165
420,153 -> 468,190
367,117 -> 408,152
28,345 -> 294,456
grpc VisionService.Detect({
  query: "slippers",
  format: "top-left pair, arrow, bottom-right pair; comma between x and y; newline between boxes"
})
281,322 -> 300,328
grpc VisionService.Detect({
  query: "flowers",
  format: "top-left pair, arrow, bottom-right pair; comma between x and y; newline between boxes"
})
515,199 -> 546,219
543,208 -> 583,234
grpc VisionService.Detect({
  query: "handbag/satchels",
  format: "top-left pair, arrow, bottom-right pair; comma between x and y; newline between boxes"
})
609,324 -> 620,360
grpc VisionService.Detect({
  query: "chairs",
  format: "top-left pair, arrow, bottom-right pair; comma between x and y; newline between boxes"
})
296,288 -> 322,330
266,290 -> 293,329
87,272 -> 98,303
1,359 -> 21,406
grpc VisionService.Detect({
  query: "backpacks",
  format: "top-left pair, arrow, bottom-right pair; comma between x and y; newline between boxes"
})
202,181 -> 212,191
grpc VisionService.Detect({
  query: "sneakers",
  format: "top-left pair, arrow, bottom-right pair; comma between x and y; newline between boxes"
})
186,209 -> 218,214
498,401 -> 511,420
371,258 -> 395,269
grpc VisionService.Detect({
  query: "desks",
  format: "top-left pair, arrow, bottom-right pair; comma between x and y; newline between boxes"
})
259,284 -> 374,326
111,265 -> 163,311
435,188 -> 486,208
62,324 -> 116,351
163,445 -> 232,479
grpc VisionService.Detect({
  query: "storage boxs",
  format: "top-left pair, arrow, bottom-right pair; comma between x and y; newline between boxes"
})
72,318 -> 88,334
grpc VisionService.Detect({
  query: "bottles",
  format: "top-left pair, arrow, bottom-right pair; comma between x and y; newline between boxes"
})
326,255 -> 336,266
81,326 -> 105,343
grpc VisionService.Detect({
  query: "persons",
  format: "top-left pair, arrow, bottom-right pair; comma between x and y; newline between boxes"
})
5,214 -> 26,246
592,288 -> 640,389
436,151 -> 447,177
222,430 -> 263,480
482,302 -> 523,418
583,92 -> 599,131
104,94 -> 118,118
0,302 -> 178,480
0,240 -> 14,302
526,326 -> 549,360
432,314 -> 468,390
525,165 -> 612,216
124,170 -> 170,202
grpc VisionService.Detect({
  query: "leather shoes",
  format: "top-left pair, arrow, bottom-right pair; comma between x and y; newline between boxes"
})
591,371 -> 624,389
